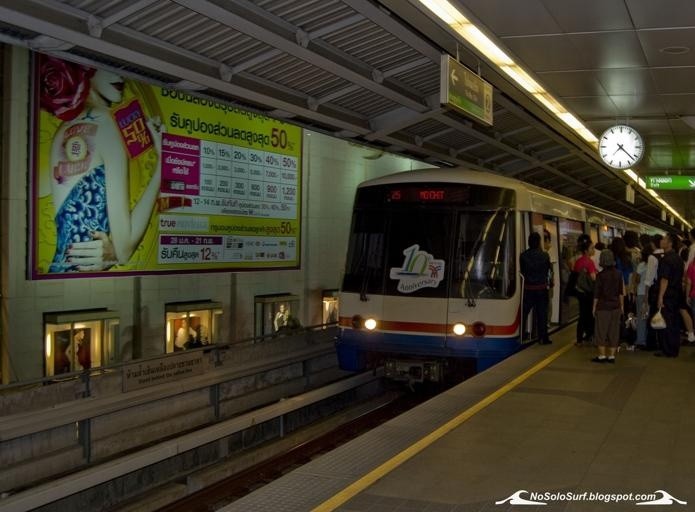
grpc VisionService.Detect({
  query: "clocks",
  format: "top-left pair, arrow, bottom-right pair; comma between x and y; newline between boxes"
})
597,117 -> 645,172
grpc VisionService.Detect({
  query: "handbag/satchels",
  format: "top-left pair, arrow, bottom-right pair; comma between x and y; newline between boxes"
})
574,266 -> 595,294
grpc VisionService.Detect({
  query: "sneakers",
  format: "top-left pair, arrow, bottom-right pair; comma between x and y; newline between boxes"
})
538,337 -> 553,345
653,350 -> 679,358
680,338 -> 695,346
590,355 -> 617,363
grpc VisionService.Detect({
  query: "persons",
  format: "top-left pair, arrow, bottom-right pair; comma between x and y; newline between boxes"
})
174,319 -> 197,350
518,231 -> 554,345
31,54 -> 168,273
64,329 -> 85,372
273,304 -> 288,330
542,225 -> 695,366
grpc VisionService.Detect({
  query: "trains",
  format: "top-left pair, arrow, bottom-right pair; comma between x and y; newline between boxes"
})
333,168 -> 669,395
